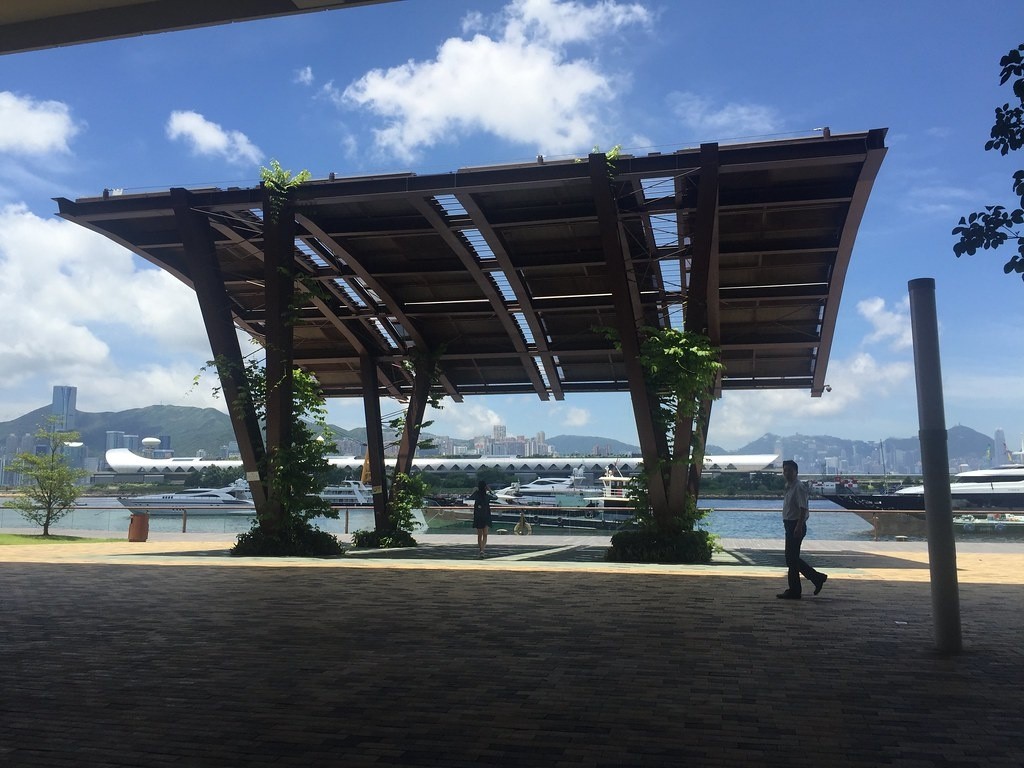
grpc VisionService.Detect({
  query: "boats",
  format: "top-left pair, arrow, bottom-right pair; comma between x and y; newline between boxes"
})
115,489 -> 256,516
420,458 -> 644,530
308,479 -> 375,506
817,464 -> 1023,532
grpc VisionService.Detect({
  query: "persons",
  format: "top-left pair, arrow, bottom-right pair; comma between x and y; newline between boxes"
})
776,460 -> 828,599
470,480 -> 498,557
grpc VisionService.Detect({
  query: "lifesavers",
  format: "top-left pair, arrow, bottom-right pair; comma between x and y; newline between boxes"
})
514,522 -> 532,536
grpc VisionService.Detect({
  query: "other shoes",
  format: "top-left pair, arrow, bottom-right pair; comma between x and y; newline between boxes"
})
813,572 -> 827,595
776,589 -> 802,599
480,551 -> 484,558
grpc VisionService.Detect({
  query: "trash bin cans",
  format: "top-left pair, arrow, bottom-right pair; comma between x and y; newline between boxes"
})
128,512 -> 150,541
